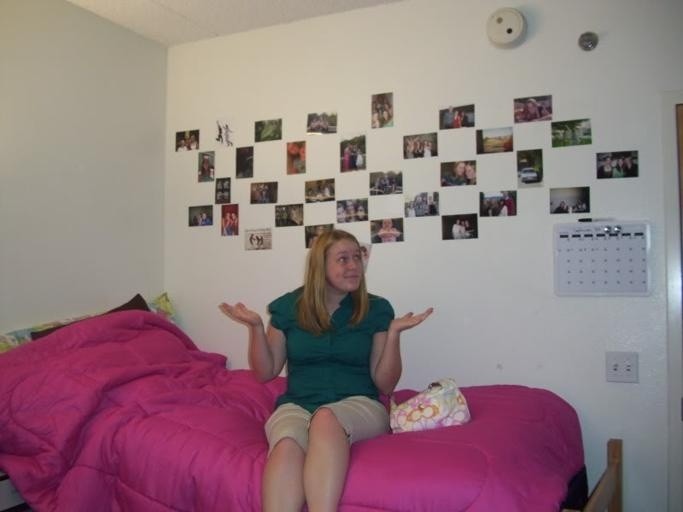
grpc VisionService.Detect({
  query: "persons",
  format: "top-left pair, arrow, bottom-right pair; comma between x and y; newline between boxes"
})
222,212 -> 238,236
371,99 -> 394,128
404,192 -> 437,216
445,162 -> 476,185
600,154 -> 638,178
452,218 -> 471,238
480,192 -> 512,216
219,227 -> 433,512
377,218 -> 401,244
517,97 -> 549,121
198,153 -> 214,179
442,104 -> 469,126
192,211 -> 210,226
308,113 -> 330,131
287,141 -> 306,175
178,129 -> 199,151
343,140 -> 363,169
336,200 -> 368,224
215,120 -> 234,146
404,137 -> 433,157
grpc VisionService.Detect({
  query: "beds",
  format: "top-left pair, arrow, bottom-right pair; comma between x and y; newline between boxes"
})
0,294 -> 623,511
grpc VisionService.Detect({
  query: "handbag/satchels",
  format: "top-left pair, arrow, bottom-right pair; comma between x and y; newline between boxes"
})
390,377 -> 471,434
